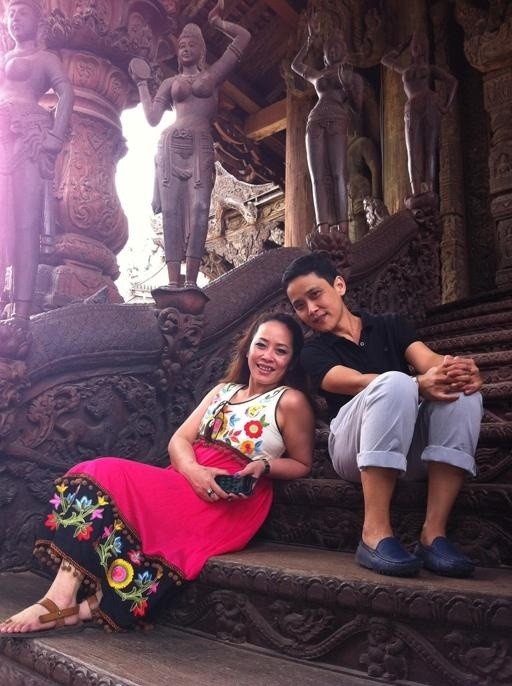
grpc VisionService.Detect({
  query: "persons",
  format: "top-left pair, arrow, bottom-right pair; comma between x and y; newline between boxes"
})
2,0 -> 72,352
1,310 -> 318,635
283,253 -> 489,579
120,0 -> 253,293
291,5 -> 364,239
381,28 -> 460,198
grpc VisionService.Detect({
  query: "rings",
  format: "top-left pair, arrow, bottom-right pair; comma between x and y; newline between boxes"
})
207,489 -> 214,497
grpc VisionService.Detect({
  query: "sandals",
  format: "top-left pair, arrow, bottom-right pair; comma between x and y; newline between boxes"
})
1,593 -> 110,639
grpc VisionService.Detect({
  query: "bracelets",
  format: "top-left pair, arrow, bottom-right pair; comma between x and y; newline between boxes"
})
410,374 -> 419,384
261,459 -> 271,475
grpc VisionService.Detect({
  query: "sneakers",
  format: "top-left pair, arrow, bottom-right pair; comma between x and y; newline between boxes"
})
353,535 -> 421,576
416,535 -> 479,579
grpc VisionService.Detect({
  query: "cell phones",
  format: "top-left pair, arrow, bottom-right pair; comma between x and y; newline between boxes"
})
214,475 -> 253,494
